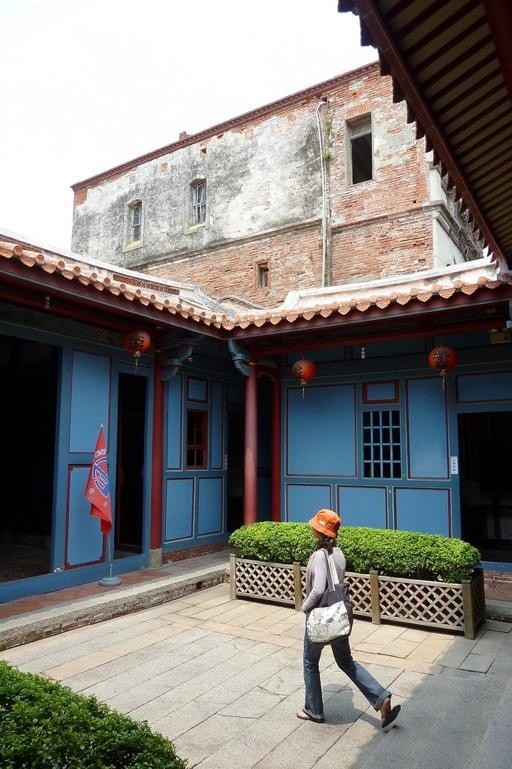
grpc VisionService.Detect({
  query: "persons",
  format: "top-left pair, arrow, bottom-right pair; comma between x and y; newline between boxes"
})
296,509 -> 400,728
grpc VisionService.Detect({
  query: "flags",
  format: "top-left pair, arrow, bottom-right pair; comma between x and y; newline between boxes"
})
86,426 -> 112,537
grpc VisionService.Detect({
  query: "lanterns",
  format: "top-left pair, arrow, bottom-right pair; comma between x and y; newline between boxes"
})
123,329 -> 151,365
428,342 -> 457,394
292,356 -> 317,399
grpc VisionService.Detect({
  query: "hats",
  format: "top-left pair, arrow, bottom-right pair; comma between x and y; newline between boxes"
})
309,509 -> 341,539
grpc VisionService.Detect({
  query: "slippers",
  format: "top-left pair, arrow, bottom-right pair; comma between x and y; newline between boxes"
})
381,704 -> 401,728
296,709 -> 325,723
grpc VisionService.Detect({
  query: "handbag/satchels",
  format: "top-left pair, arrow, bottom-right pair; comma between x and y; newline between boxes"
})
305,550 -> 353,647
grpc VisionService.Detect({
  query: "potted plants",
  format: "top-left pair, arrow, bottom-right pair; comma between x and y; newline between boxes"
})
219,519 -> 490,639
